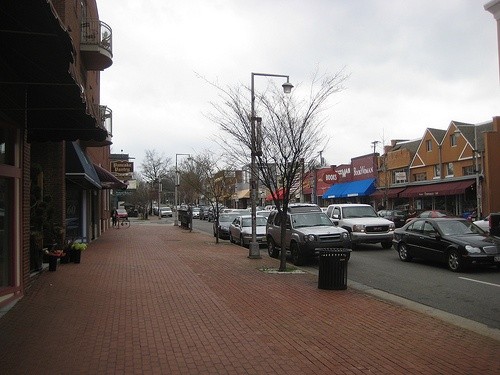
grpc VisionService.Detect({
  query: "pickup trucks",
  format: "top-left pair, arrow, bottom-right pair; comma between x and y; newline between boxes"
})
321,203 -> 396,249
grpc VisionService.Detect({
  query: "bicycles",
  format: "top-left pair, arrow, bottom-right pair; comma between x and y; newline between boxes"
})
116,216 -> 131,229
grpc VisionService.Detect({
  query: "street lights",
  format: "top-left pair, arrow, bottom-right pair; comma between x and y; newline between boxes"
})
454,125 -> 481,221
174,153 -> 192,226
248,73 -> 295,259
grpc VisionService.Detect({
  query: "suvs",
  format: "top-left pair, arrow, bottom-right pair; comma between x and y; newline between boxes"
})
266,204 -> 353,266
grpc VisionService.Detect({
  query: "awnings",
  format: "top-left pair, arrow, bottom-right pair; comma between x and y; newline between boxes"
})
0,0 -> 128,190
228,176 -> 476,200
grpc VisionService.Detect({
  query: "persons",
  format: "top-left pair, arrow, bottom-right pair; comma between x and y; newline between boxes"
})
110,207 -> 118,226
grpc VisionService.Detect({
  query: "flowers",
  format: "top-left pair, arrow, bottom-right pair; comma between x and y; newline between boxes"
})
71,243 -> 87,250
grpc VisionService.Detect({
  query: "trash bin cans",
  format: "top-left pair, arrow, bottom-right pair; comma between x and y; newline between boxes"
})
315,248 -> 352,290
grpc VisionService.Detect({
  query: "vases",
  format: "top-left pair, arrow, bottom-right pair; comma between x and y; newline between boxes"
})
70,250 -> 81,263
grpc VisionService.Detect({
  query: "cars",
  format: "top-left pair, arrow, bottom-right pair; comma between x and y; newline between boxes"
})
175,203 -> 339,251
377,208 -> 500,272
116,209 -> 128,220
149,201 -> 172,217
